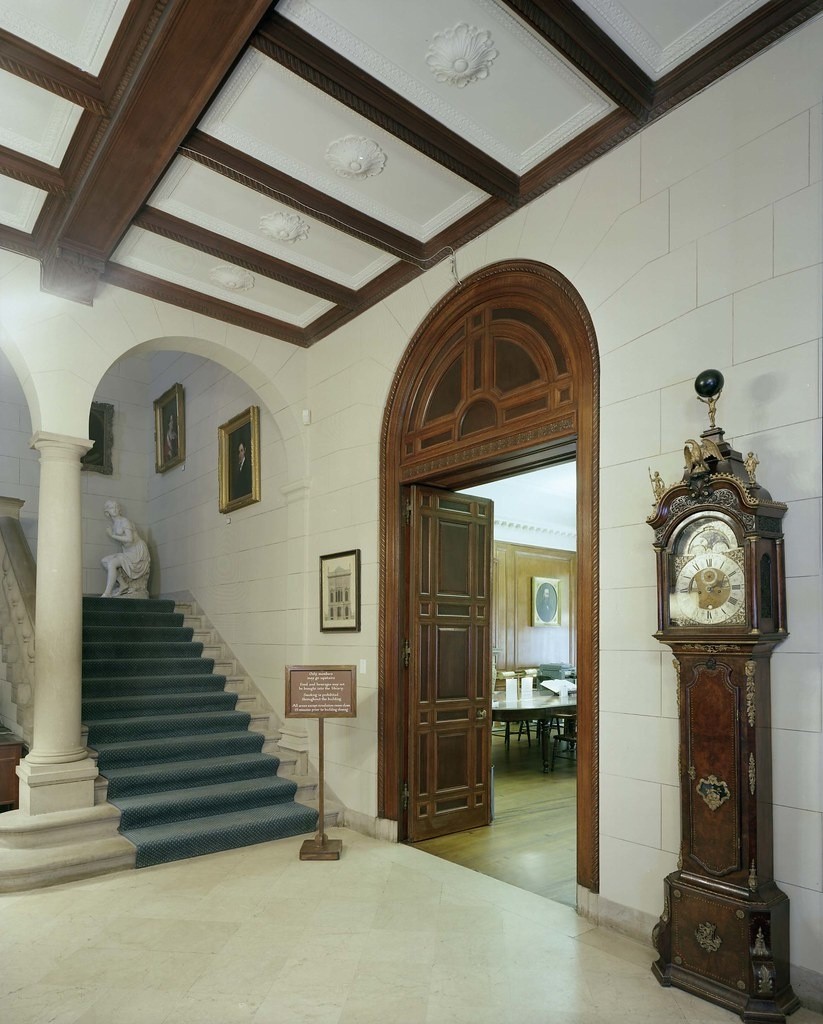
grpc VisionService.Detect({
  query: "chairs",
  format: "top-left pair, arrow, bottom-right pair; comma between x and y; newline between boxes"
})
550,731 -> 577,772
490,671 -> 532,752
518,669 -> 561,748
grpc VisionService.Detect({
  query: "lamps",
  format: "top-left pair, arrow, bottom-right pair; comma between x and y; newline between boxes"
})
423,21 -> 499,89
260,211 -> 310,244
210,266 -> 255,292
325,137 -> 387,181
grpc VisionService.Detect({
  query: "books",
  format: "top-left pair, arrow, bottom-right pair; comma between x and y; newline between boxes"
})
540,678 -> 577,695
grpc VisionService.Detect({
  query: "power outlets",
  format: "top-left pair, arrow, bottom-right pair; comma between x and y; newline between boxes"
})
302,409 -> 311,424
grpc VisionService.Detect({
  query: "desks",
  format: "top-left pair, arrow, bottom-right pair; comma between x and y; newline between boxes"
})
493,688 -> 577,774
0,725 -> 24,810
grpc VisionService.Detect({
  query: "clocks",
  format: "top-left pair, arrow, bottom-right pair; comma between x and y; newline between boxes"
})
646,370 -> 790,640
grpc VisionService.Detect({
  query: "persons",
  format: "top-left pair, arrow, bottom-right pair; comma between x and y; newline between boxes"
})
166,415 -> 176,457
538,588 -> 554,621
100,499 -> 150,597
234,441 -> 251,496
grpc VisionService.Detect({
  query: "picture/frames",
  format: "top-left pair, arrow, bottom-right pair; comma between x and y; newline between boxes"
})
81,402 -> 115,475
153,382 -> 186,473
218,407 -> 261,514
531,576 -> 563,627
319,550 -> 361,634
